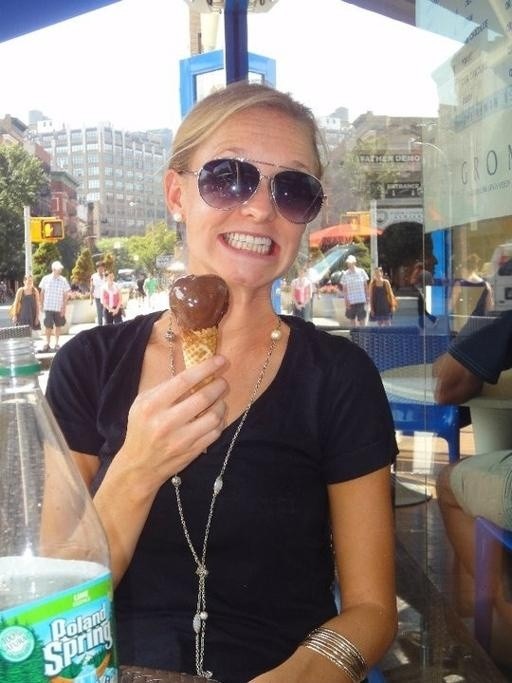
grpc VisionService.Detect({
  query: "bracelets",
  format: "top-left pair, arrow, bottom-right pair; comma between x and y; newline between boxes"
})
300,626 -> 370,682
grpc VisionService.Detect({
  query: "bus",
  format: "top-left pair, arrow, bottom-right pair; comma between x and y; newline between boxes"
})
115,268 -> 138,291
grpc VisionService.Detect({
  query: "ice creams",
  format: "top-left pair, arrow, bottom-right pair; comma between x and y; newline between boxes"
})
169,274 -> 229,455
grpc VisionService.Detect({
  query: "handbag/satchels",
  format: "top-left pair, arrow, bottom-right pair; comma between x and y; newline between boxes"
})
387,294 -> 397,311
9,302 -> 21,315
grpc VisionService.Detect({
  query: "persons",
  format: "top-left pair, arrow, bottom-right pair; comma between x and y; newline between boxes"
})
489,241 -> 512,312
135,270 -> 176,310
10,273 -> 41,331
290,265 -> 313,319
447,251 -> 494,340
412,262 -> 440,329
429,254 -> 512,618
89,259 -> 108,326
366,265 -> 396,327
339,254 -> 370,327
38,82 -> 402,683
101,269 -> 125,324
37,259 -> 70,352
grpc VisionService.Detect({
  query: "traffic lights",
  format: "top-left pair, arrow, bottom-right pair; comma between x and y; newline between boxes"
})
39,218 -> 65,240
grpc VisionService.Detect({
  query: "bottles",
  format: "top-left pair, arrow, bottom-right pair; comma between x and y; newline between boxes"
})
1,336 -> 121,682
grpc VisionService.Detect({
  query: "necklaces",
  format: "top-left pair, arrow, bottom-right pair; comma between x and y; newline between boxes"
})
166,308 -> 282,678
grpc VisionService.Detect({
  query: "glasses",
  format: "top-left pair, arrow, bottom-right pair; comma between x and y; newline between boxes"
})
185,158 -> 327,225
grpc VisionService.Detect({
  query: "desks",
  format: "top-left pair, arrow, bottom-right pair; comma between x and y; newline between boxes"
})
379,362 -> 511,459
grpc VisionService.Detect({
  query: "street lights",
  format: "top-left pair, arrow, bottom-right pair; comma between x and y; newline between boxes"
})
413,140 -> 455,310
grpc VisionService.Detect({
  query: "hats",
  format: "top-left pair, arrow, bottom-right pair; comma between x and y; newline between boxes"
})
51,261 -> 64,269
346,255 -> 358,265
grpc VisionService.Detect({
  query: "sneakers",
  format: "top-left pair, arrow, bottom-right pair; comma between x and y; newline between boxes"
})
432,317 -> 441,329
42,343 -> 59,352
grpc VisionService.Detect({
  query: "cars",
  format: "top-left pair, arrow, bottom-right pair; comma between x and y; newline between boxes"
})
296,242 -> 372,292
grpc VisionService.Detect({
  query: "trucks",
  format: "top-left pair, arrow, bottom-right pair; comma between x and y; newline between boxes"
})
477,242 -> 512,324
